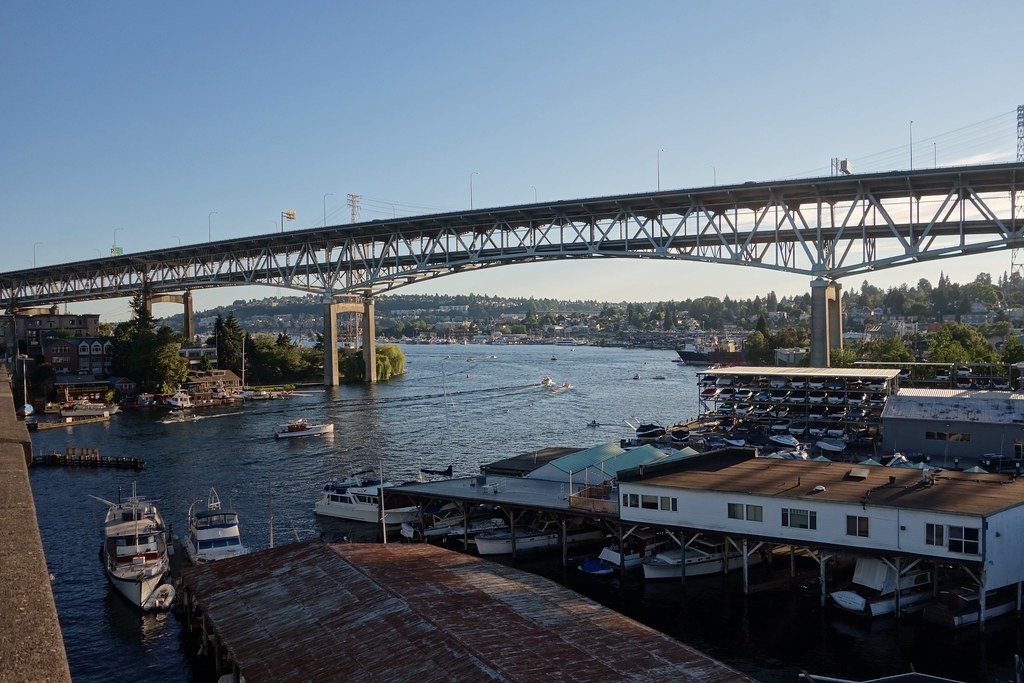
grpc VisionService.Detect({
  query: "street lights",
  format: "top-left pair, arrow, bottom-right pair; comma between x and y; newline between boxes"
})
910,120 -> 914,171
323,193 -> 336,226
933,143 -> 937,169
470,172 -> 481,212
657,146 -> 664,192
530,185 -> 538,203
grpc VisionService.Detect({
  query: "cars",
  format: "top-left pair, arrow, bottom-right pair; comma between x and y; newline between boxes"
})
980,452 -> 1021,473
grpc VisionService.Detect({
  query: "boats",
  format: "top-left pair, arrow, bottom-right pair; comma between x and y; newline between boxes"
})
577,526 -> 694,574
184,485 -> 250,565
636,365 -> 1024,453
312,467 -> 423,524
143,583 -> 175,610
87,480 -> 169,607
642,532 -> 793,578
475,509 -> 614,554
271,332 -> 755,365
826,557 -> 957,616
43,380 -> 288,424
400,498 -> 501,537
924,582 -> 1020,627
274,419 -> 334,438
448,506 -> 542,543
